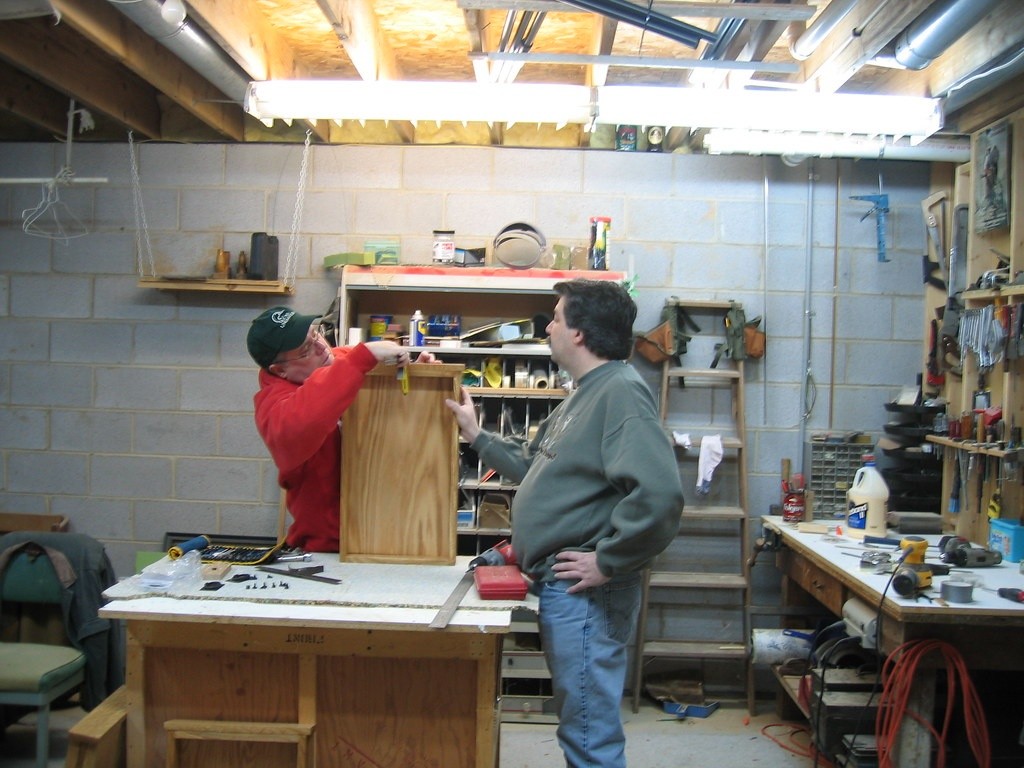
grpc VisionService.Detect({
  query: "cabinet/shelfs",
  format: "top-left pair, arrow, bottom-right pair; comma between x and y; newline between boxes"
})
925,109 -> 1024,519
335,264 -> 628,558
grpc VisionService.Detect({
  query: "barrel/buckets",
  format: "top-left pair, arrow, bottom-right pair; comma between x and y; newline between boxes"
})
846,462 -> 889,539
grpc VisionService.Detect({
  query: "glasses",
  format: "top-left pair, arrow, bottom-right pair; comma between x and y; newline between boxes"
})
271,324 -> 324,364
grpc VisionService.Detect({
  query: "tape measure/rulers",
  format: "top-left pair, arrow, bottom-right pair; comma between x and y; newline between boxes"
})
428,571 -> 474,629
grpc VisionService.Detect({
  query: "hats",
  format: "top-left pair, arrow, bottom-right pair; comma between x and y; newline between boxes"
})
247,307 -> 322,370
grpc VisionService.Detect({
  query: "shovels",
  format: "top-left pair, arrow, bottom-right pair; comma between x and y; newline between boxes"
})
663,700 -> 720,718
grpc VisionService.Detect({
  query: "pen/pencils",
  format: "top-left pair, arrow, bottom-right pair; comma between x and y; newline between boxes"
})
782,480 -> 793,493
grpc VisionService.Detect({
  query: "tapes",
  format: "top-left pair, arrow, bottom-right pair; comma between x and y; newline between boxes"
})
940,581 -> 974,603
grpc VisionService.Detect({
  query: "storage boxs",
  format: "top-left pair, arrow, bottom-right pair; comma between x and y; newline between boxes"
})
481,321 -> 521,342
478,492 -> 510,530
456,490 -> 476,530
472,565 -> 528,601
988,519 -> 1024,564
814,691 -> 895,758
810,667 -> 888,725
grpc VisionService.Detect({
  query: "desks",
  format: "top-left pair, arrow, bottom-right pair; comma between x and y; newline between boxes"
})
96,554 -> 511,767
761,514 -> 1024,768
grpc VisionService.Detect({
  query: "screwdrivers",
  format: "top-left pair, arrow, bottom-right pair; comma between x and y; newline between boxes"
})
983,587 -> 1024,602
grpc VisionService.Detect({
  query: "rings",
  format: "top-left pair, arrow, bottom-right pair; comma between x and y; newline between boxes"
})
399,351 -> 406,356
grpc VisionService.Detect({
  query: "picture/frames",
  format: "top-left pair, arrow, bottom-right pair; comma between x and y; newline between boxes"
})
971,121 -> 1010,232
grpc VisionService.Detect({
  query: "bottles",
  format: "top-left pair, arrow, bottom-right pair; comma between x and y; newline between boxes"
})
432,230 -> 456,268
408,309 -> 426,346
586,216 -> 612,271
217,250 -> 246,274
647,126 -> 666,151
783,489 -> 805,523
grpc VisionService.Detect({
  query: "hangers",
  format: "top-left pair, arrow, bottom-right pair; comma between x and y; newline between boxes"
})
21,181 -> 87,248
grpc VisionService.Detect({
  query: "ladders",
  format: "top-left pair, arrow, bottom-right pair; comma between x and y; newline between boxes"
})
631,295 -> 760,719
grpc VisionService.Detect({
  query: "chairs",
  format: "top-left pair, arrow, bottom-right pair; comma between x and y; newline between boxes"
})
0,510 -> 86,768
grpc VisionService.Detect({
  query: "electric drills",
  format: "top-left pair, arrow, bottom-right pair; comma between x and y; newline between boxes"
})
939,535 -> 1002,567
892,536 -> 974,595
464,539 -> 516,576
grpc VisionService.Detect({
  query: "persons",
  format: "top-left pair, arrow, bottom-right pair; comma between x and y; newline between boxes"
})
247,306 -> 443,552
444,277 -> 684,768
